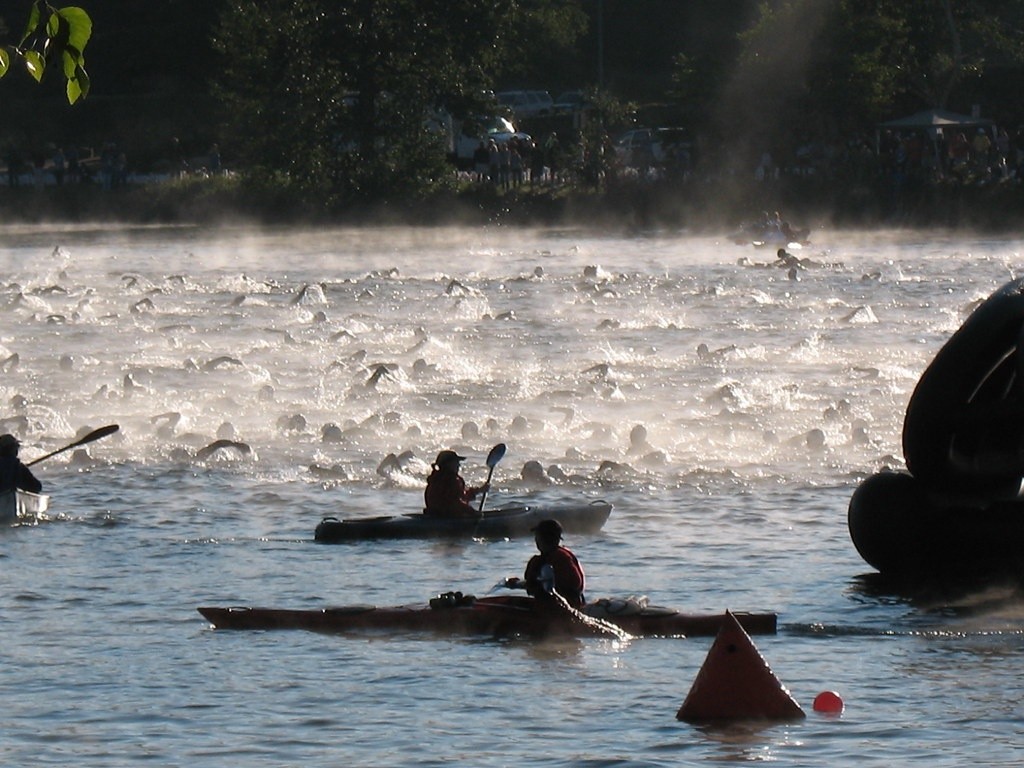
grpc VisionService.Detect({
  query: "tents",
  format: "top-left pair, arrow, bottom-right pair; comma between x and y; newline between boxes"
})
873,107 -> 999,159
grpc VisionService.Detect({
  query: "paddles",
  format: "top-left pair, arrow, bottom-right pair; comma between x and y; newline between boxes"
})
479,443 -> 506,511
489,564 -> 556,593
24,424 -> 119,467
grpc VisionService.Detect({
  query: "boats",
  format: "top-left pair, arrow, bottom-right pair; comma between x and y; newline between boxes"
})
195,602 -> 780,637
0,486 -> 50,523
314,497 -> 613,543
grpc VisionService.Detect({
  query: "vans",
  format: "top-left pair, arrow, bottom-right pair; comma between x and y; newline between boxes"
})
494,91 -> 555,115
608,128 -> 685,165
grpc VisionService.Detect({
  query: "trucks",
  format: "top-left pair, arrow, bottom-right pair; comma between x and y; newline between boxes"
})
434,105 -> 534,170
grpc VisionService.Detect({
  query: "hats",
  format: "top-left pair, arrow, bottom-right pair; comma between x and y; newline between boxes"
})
436,451 -> 466,464
530,519 -> 562,534
0,434 -> 23,447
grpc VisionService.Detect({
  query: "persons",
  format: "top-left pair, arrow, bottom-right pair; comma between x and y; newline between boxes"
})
0,433 -> 42,493
0,99 -> 1024,487
423,451 -> 491,521
505,519 -> 587,611
